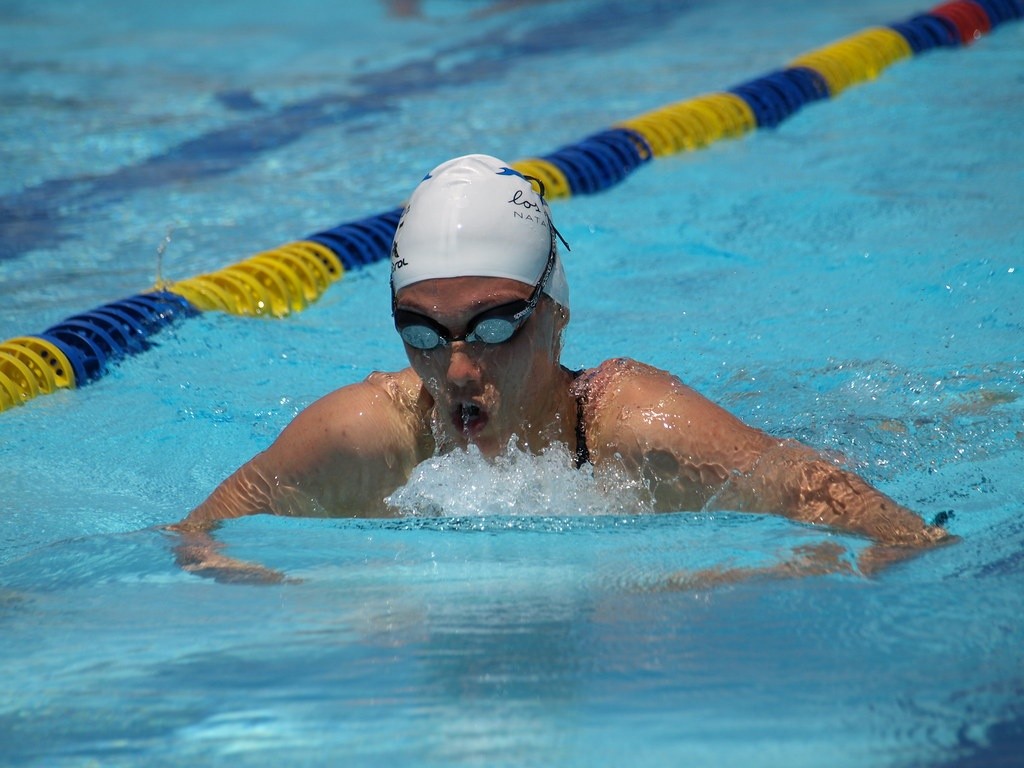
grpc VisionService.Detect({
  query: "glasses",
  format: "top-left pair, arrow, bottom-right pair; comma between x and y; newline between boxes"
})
393,298 -> 532,353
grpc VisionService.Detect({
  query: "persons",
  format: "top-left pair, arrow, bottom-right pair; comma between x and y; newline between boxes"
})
171,155 -> 963,545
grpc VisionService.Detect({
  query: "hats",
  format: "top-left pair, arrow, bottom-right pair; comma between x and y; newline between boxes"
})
390,154 -> 571,325
